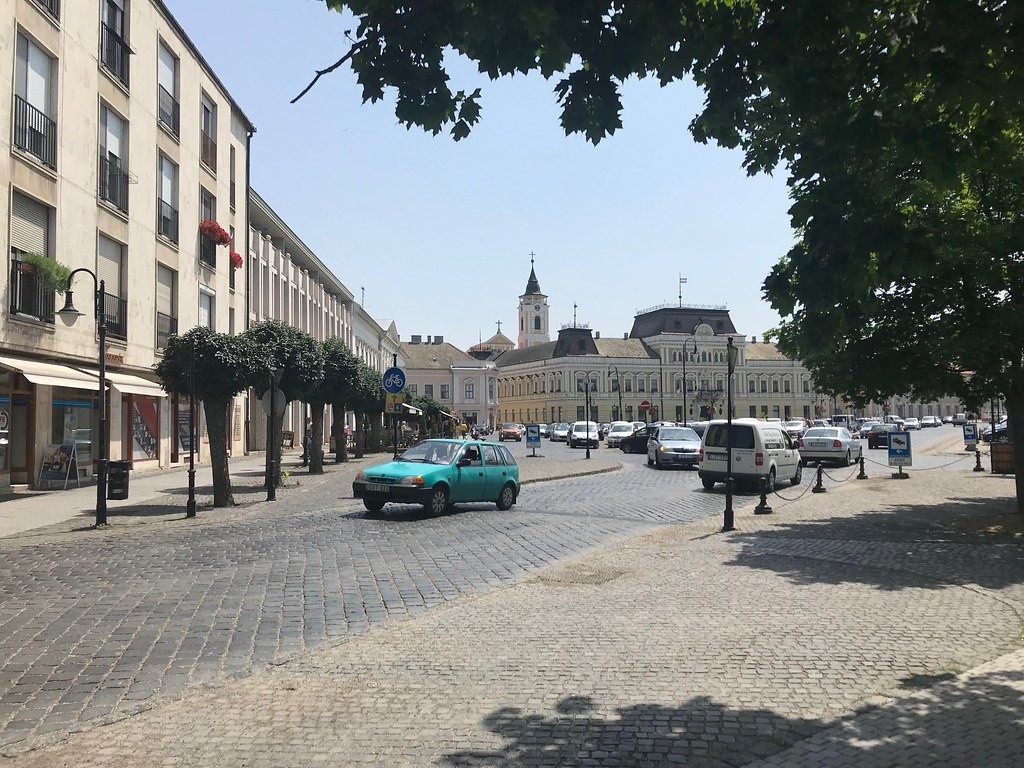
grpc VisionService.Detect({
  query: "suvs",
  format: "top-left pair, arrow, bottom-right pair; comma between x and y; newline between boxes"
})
792,417 -> 814,428
499,422 -> 522,442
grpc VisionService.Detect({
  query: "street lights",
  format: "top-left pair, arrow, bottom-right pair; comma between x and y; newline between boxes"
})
584,373 -> 591,459
51,268 -> 110,526
683,338 -> 700,427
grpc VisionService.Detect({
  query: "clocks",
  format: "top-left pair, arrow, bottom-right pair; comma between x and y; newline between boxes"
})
533,303 -> 542,314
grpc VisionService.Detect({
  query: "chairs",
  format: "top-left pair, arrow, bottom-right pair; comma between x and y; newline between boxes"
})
435,446 -> 447,460
464,449 -> 477,461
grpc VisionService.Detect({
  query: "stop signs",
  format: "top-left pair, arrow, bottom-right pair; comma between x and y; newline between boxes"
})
641,400 -> 650,410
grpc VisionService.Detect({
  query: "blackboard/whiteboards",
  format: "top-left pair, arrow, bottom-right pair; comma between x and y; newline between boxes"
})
281,431 -> 295,447
40,442 -> 80,480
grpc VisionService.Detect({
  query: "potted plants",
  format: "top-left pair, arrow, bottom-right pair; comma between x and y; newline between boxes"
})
20,250 -> 80,296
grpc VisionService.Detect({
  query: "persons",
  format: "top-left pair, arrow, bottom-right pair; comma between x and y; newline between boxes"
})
455,423 -> 461,439
401,421 -> 411,447
460,422 -> 468,440
299,418 -> 313,460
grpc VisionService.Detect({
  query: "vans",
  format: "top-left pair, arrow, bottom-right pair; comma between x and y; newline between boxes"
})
832,415 -> 858,432
571,420 -> 600,449
855,418 -> 872,430
812,419 -> 832,427
610,421 -> 646,433
698,420 -> 803,493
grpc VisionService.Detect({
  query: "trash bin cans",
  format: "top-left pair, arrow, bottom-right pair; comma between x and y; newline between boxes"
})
108,459 -> 130,500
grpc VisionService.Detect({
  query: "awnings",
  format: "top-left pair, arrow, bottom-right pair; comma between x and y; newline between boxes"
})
77,367 -> 169,396
0,353 -> 110,391
402,403 -> 422,415
439,410 -> 459,423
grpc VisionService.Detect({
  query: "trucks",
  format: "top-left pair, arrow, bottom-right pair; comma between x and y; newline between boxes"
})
865,415 -> 904,424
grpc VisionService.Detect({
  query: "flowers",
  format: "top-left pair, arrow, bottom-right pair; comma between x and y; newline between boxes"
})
201,219 -> 243,270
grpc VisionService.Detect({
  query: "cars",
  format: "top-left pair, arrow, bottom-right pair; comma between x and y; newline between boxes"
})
566,423 -> 574,445
353,438 -> 520,517
921,416 -> 943,428
536,424 -> 548,436
619,426 -> 658,454
860,421 -> 881,436
799,427 -> 862,467
647,426 -> 702,469
596,422 -> 611,440
903,418 -> 920,430
546,422 -> 571,441
942,416 -> 953,424
784,421 -> 809,439
983,416 -> 1009,443
867,425 -> 899,449
514,424 -> 527,435
474,425 -> 488,435
652,420 -> 710,440
607,424 -> 632,447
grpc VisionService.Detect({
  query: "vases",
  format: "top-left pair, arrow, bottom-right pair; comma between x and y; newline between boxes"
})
201,227 -> 214,238
